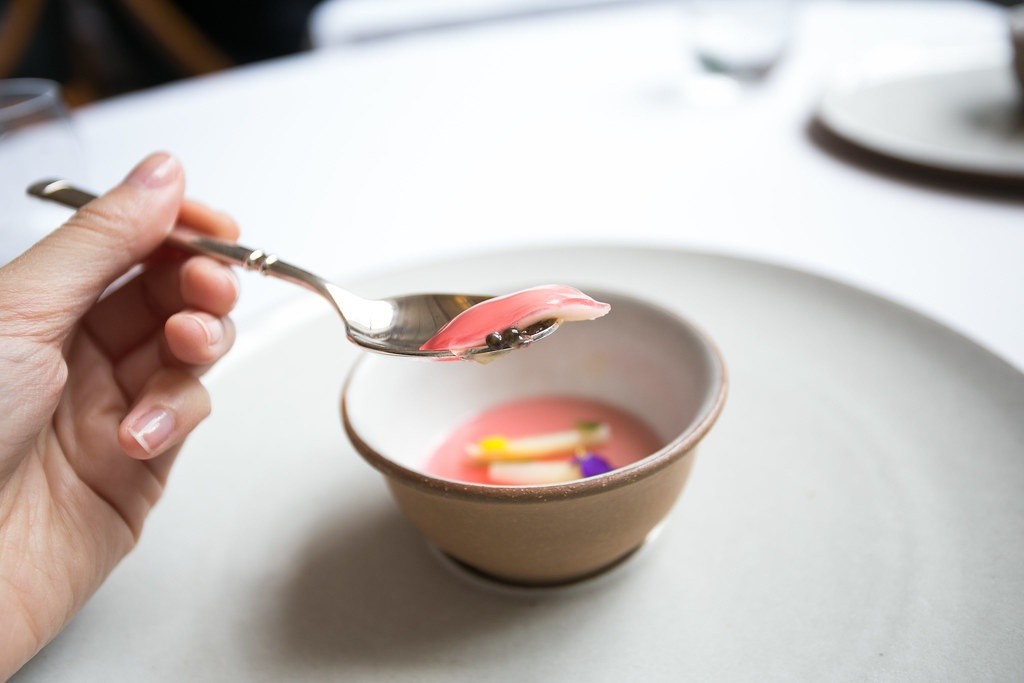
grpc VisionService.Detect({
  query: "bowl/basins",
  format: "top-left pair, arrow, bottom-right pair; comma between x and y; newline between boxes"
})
336,284 -> 731,595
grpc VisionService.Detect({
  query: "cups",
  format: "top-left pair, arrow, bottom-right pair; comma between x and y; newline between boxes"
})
0,77 -> 86,215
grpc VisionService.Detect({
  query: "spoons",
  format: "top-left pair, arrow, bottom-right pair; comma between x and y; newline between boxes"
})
25,174 -> 564,363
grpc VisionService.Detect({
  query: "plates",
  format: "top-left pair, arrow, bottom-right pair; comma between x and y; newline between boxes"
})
811,68 -> 1024,176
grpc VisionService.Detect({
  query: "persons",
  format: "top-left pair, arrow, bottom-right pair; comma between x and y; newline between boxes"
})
0,150 -> 241,681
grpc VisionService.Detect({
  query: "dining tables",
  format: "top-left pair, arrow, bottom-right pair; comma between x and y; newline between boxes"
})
0,0 -> 1022,681
